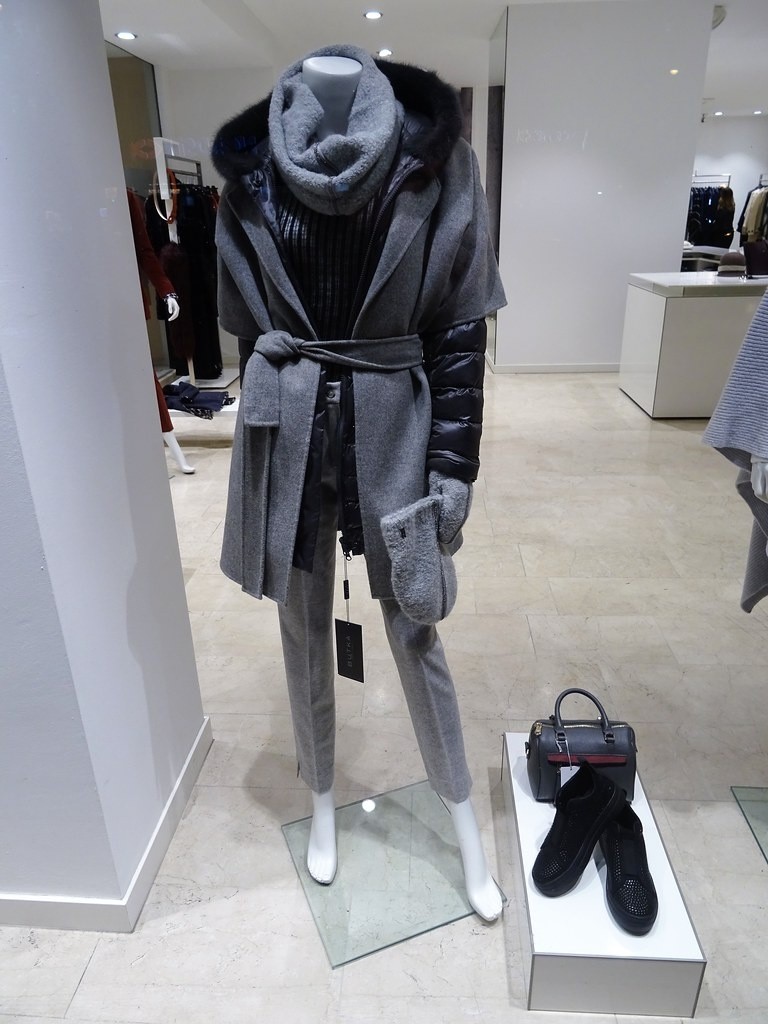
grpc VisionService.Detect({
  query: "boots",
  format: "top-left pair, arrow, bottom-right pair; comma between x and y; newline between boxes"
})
532,755 -> 658,936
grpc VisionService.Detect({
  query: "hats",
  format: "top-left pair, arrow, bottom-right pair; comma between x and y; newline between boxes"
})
381,494 -> 458,626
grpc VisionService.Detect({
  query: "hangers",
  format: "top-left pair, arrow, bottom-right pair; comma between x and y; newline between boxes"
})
148,169 -> 217,196
757,179 -> 768,188
691,180 -> 728,193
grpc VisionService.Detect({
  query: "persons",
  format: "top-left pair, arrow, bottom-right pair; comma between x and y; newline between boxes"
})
211,45 -> 504,921
127,188 -> 196,473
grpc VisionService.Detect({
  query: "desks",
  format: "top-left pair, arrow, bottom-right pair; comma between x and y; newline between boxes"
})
618,272 -> 768,419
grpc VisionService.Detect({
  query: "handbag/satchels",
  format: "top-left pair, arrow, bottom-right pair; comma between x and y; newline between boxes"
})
525,688 -> 638,805
